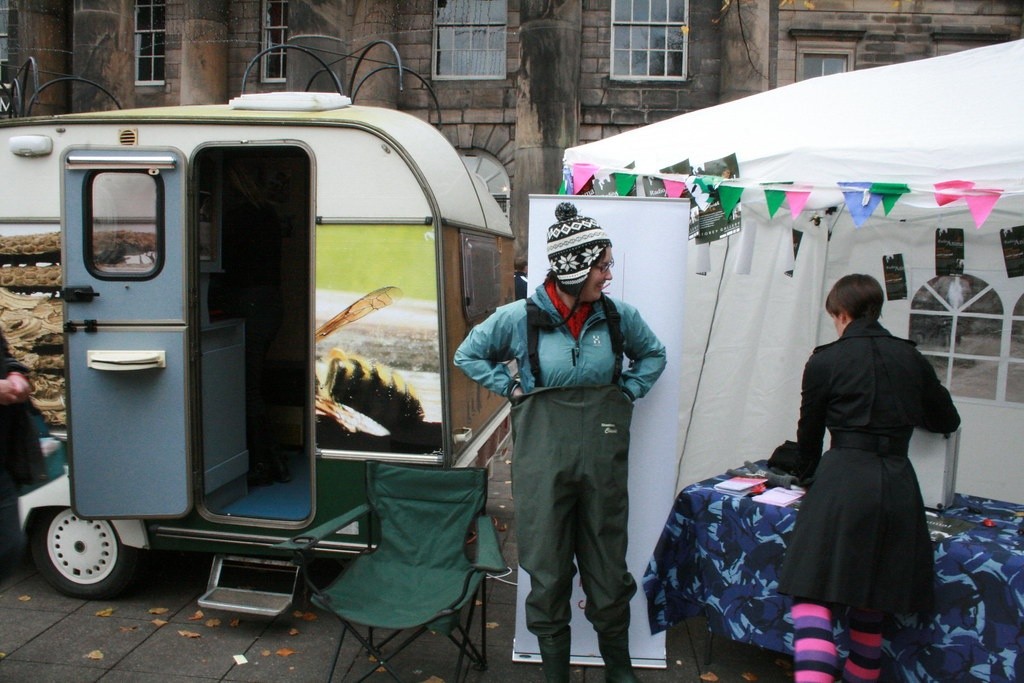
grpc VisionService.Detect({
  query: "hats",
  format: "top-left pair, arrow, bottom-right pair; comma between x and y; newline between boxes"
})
530,202 -> 612,330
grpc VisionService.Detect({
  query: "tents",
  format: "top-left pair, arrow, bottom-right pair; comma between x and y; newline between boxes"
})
562,36 -> 1024,669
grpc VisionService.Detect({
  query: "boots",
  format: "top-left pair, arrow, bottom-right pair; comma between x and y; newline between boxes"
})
538,624 -> 571,683
598,628 -> 642,683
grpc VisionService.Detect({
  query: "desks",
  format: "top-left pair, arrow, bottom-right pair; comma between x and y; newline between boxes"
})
640,458 -> 1024,682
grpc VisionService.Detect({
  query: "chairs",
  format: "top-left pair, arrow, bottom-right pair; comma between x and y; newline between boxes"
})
271,464 -> 510,683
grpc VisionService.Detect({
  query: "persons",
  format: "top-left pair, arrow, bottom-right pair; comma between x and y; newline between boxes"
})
0,326 -> 50,580
454,201 -> 668,683
514,256 -> 528,301
795,274 -> 962,683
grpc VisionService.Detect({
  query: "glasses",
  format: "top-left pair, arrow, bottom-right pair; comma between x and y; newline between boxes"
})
591,260 -> 612,273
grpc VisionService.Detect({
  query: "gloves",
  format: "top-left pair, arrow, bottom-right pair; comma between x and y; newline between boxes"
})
727,461 -> 799,490
